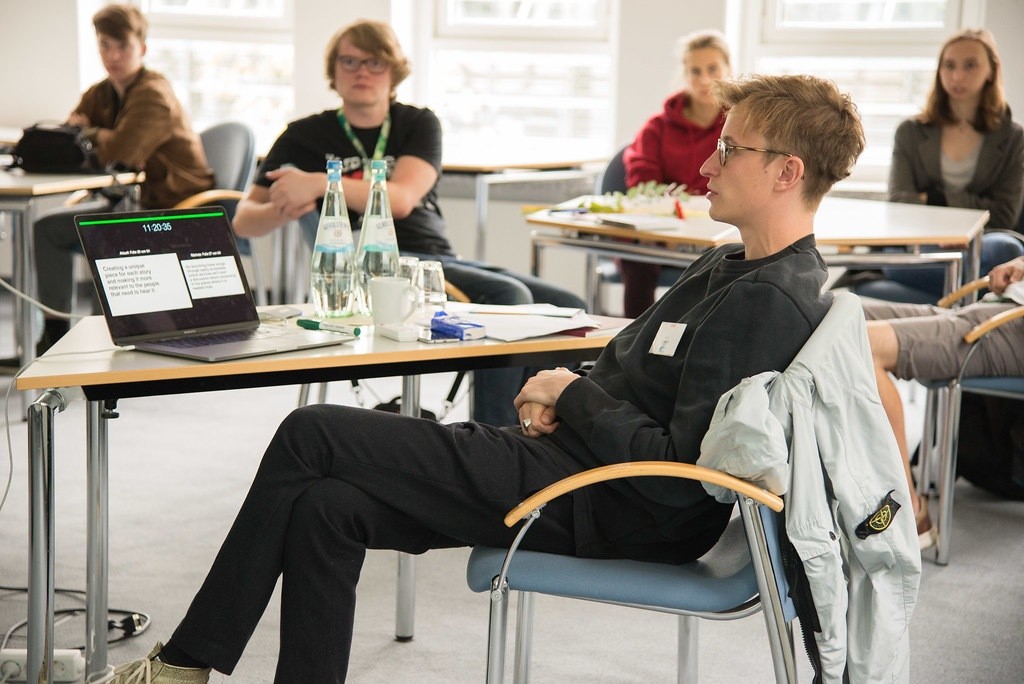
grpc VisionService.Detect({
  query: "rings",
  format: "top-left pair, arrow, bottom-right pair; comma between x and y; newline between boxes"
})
522,419 -> 531,430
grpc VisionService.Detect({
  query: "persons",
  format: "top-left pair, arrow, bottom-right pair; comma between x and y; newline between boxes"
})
232,19 -> 588,425
615,31 -> 734,319
32,3 -> 218,358
100,74 -> 865,684
883,28 -> 1024,292
860,255 -> 1024,551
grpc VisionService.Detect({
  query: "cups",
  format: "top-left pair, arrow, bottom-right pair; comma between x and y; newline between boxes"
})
397,255 -> 419,281
371,277 -> 419,329
413,261 -> 446,324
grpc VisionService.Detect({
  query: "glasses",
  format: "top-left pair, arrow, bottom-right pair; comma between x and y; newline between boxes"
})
717,139 -> 806,183
334,53 -> 389,74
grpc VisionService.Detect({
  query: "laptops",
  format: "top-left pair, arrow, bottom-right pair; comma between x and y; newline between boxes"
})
73,206 -> 355,362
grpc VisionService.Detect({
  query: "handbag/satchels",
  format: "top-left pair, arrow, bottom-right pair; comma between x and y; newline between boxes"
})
3,121 -> 106,174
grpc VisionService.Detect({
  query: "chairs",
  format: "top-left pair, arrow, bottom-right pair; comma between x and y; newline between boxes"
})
467,289 -> 865,684
278,164 -> 465,409
587,147 -> 671,319
200,123 -> 256,214
919,276 -> 1024,565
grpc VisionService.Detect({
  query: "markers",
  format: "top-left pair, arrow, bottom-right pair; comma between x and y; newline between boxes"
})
297,319 -> 361,337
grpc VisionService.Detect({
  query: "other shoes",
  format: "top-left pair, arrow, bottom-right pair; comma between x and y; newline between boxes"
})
105,641 -> 212,684
0,339 -> 57,376
914,496 -> 938,551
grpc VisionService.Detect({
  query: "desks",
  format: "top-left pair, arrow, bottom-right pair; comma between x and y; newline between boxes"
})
527,195 -> 990,562
16,305 -> 637,684
0,171 -> 146,421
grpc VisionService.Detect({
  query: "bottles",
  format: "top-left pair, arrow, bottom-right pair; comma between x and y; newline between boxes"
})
311,160 -> 356,317
353,161 -> 401,314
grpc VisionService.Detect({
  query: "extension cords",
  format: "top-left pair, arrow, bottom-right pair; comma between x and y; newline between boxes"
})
0,648 -> 84,682
